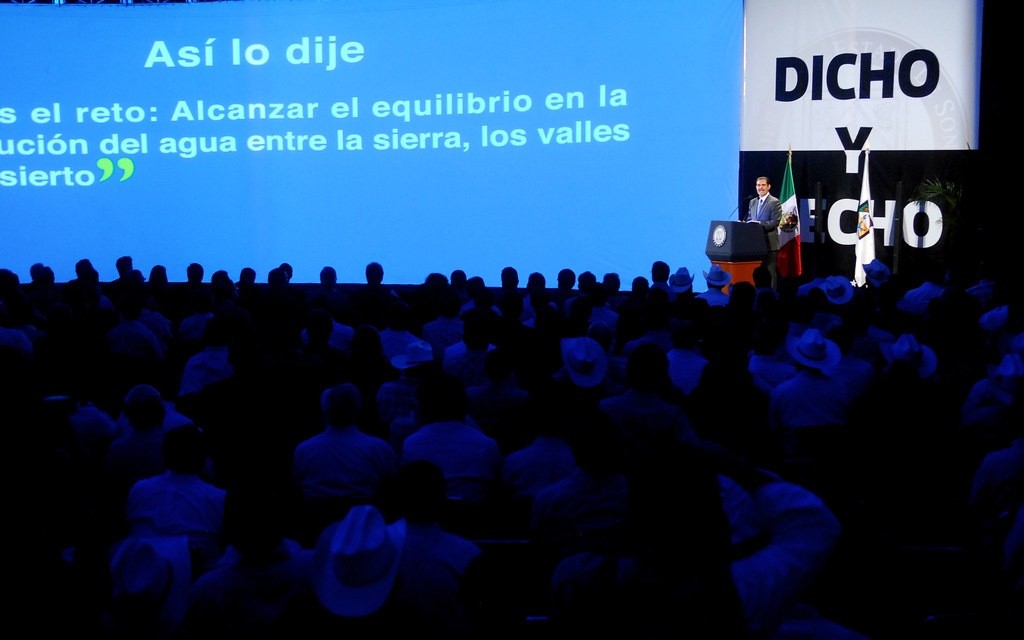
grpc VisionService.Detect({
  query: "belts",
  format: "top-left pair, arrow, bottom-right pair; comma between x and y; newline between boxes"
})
759,199 -> 763,215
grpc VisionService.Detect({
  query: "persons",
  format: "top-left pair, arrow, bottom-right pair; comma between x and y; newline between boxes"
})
0,254 -> 1018,639
746,176 -> 782,292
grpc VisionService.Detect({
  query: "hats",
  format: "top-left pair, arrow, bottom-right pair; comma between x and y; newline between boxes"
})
391,341 -> 433,370
98,534 -> 193,640
862,259 -> 891,287
898,281 -> 946,318
669,267 -> 695,293
788,328 -> 840,371
820,275 -> 854,304
314,504 -> 411,619
881,333 -> 937,377
702,265 -> 731,286
560,336 -> 607,387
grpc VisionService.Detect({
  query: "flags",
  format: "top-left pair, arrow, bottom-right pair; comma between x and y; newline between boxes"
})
854,148 -> 876,289
776,151 -> 803,278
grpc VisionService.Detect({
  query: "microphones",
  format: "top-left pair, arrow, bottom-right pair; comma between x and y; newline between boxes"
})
728,195 -> 753,218
